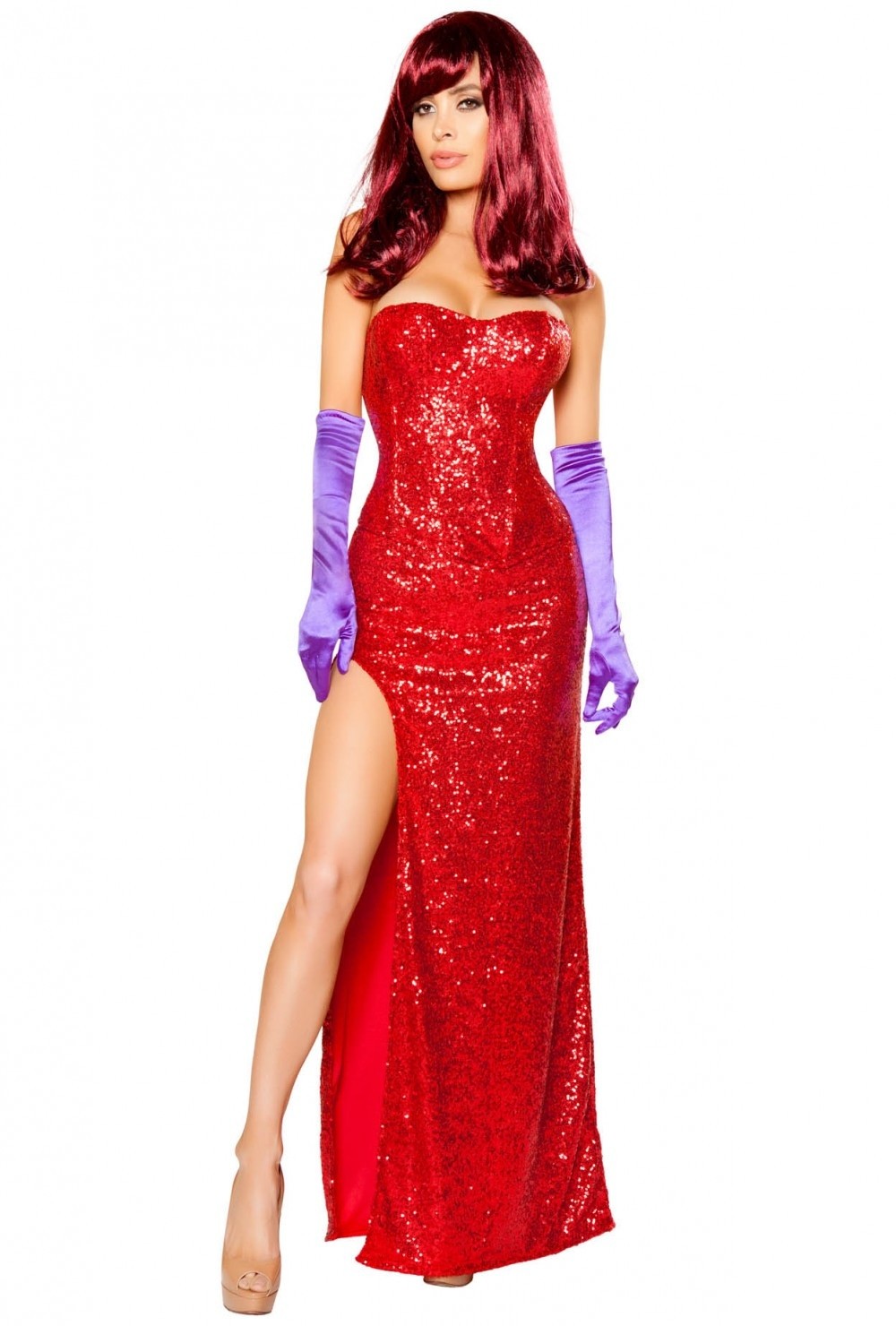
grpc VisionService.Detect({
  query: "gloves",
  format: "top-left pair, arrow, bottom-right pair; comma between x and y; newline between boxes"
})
548,441 -> 640,735
298,408 -> 367,703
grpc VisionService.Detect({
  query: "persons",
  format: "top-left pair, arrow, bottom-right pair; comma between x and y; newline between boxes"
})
225,13 -> 640,1317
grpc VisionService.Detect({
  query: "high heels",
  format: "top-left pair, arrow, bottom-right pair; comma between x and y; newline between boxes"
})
220,1161 -> 285,1315
423,1273 -> 474,1288
